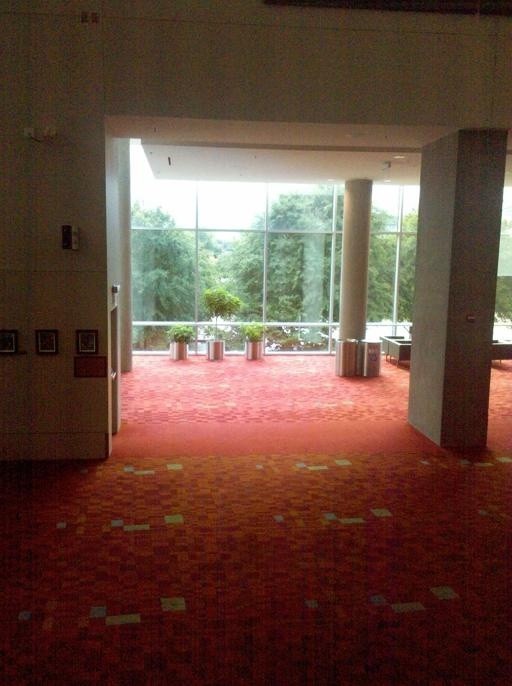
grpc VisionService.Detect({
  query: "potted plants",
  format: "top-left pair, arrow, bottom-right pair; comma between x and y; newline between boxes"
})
243,320 -> 265,361
203,286 -> 241,361
165,325 -> 195,361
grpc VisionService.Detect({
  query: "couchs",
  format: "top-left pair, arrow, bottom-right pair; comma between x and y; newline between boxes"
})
490,343 -> 512,365
493,340 -> 499,343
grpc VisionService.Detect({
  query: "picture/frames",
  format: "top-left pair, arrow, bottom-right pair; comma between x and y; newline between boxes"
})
0,329 -> 19,355
75,328 -> 100,356
35,328 -> 59,356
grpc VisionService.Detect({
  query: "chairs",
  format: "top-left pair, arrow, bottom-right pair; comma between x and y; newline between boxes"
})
388,340 -> 411,367
379,335 -> 407,362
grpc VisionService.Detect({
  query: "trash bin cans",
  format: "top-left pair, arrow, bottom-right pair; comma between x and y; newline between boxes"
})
335,338 -> 380,377
